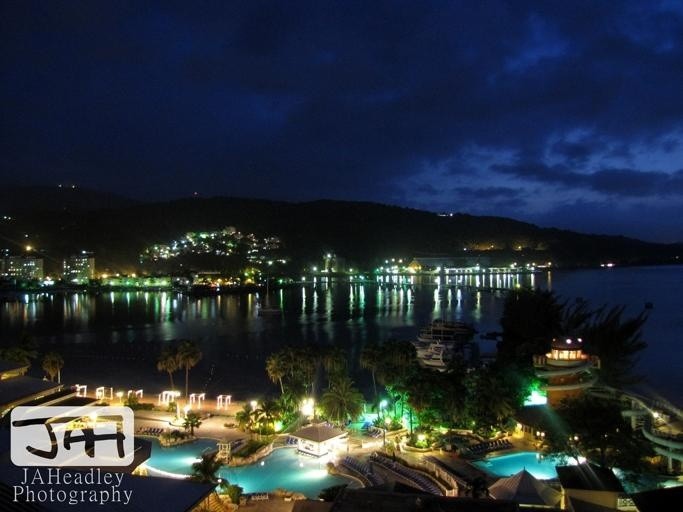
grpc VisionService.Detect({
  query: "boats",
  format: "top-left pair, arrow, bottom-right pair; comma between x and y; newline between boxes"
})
416,341 -> 450,373
416,319 -> 459,344
258,304 -> 288,316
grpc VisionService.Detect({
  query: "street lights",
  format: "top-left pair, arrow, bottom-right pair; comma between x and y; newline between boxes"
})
380,400 -> 388,445
653,412 -> 673,473
117,392 -> 123,403
